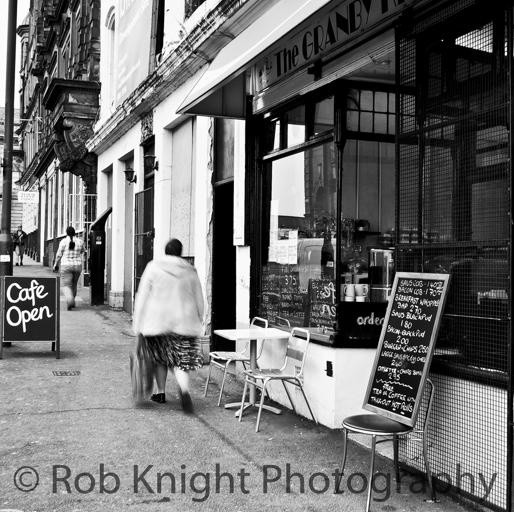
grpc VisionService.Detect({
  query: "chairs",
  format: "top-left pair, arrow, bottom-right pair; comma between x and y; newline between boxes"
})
333,377 -> 434,512
203,316 -> 268,407
238,326 -> 318,433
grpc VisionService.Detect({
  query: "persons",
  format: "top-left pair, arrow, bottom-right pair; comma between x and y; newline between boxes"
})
11,225 -> 28,266
129,235 -> 206,414
49,225 -> 85,312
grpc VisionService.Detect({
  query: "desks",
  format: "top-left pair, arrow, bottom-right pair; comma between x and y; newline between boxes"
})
213,327 -> 291,417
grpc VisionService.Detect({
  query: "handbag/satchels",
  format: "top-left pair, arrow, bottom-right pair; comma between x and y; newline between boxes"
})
129,334 -> 153,402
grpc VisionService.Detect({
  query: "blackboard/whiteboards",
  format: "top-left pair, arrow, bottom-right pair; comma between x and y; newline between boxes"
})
361,271 -> 451,428
259,265 -> 339,333
3,276 -> 61,342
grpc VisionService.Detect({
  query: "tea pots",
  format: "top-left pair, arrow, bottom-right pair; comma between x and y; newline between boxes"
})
357,220 -> 370,232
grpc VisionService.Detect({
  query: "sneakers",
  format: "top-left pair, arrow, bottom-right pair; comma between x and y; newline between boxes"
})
151,393 -> 165,403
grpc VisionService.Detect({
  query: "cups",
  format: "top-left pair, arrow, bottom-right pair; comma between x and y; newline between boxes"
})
354,284 -> 369,296
343,284 -> 354,296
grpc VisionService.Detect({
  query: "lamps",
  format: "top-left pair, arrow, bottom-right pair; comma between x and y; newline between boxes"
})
123,165 -> 136,183
144,152 -> 158,171
